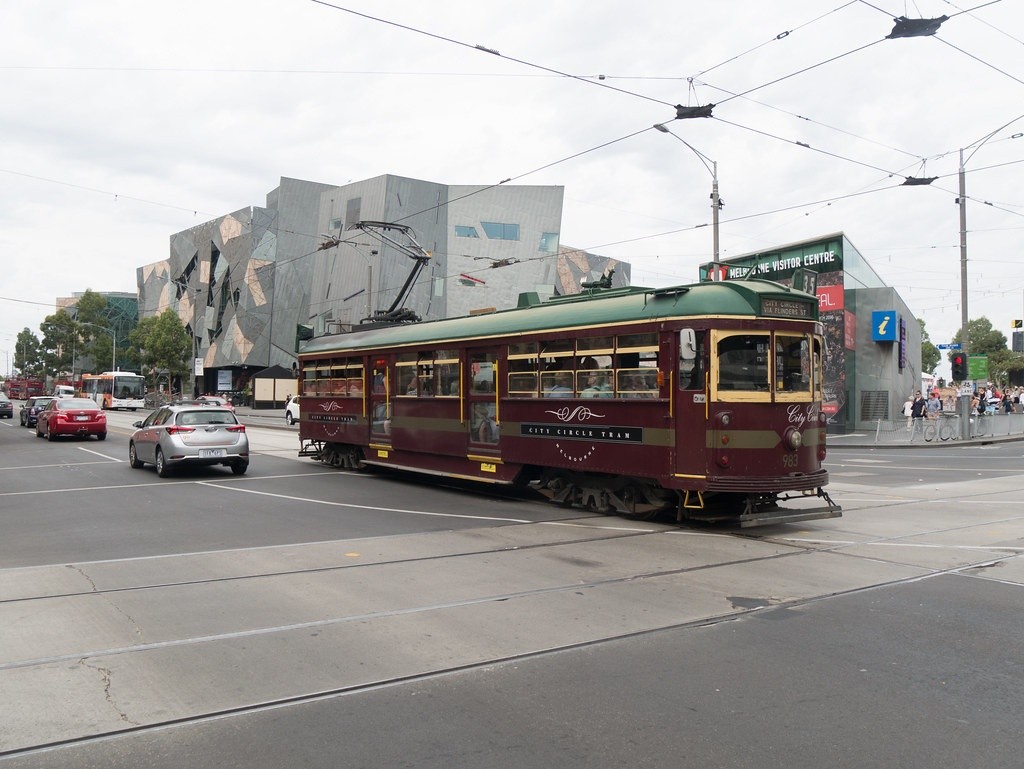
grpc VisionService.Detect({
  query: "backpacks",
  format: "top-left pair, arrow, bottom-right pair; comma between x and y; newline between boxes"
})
975,399 -> 986,413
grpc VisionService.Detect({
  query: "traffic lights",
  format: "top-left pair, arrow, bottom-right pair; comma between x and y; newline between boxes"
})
951,352 -> 968,381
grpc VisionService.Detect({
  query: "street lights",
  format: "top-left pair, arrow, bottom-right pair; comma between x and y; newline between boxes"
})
654,122 -> 719,283
156,275 -> 197,399
79,322 -> 116,371
43,322 -> 76,373
4,338 -> 26,372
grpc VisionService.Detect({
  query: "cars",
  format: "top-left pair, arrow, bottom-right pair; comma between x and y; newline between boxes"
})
192,395 -> 236,415
0,391 -> 13,419
19,396 -> 62,427
285,395 -> 300,427
129,398 -> 250,478
35,398 -> 108,441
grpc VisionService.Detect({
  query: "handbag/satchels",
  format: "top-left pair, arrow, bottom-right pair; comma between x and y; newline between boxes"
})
1011,405 -> 1015,410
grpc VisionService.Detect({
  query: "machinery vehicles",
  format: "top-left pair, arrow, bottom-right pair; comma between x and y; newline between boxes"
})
53,369 -> 81,391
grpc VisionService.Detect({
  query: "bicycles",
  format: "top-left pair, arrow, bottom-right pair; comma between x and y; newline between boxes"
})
922,412 -> 988,443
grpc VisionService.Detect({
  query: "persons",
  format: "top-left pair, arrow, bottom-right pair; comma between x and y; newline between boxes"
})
305,375 -> 363,397
223,392 -> 230,401
972,386 -> 1024,415
513,355 -> 658,398
902,390 -> 942,433
285,394 -> 292,411
374,368 -> 498,442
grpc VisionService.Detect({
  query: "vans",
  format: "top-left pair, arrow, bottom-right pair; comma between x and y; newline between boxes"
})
53,385 -> 74,399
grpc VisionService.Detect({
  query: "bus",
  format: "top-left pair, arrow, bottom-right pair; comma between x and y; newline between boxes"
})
295,260 -> 843,530
18,373 -> 46,400
4,377 -> 21,399
79,372 -> 145,411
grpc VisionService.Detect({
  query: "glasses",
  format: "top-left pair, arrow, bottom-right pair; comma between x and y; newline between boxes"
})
916,393 -> 920,395
